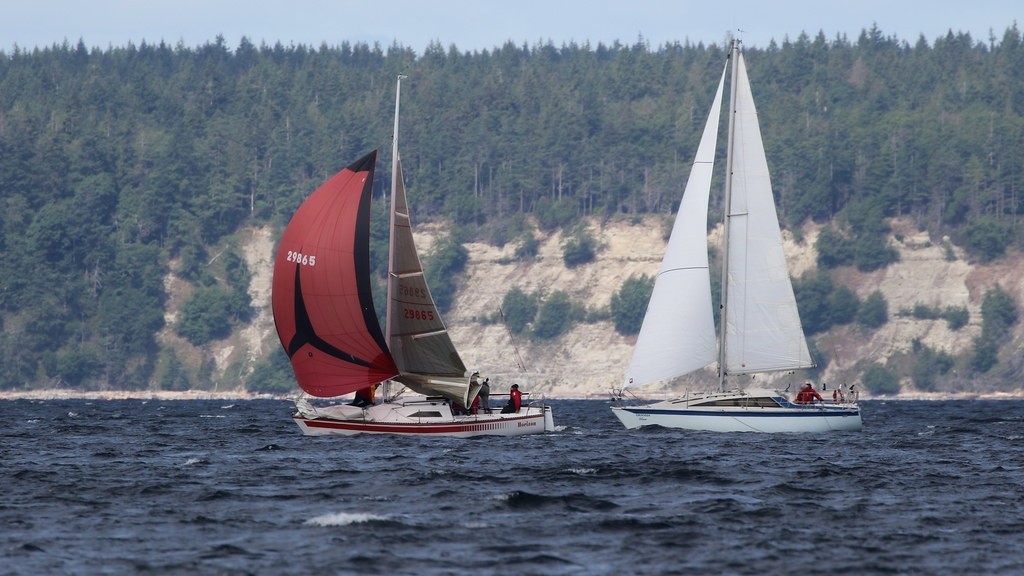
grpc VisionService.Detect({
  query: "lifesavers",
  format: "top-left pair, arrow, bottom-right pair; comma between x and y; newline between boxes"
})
831,390 -> 844,404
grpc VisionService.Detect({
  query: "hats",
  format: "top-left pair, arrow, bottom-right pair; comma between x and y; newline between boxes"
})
511,384 -> 518,389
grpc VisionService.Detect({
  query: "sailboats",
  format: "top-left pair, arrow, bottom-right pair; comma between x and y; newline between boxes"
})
608,29 -> 864,433
270,74 -> 556,438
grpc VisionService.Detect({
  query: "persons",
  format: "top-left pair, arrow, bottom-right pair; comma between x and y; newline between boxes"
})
477,382 -> 493,414
346,381 -> 382,408
500,384 -> 522,414
452,394 -> 480,416
795,383 -> 825,404
